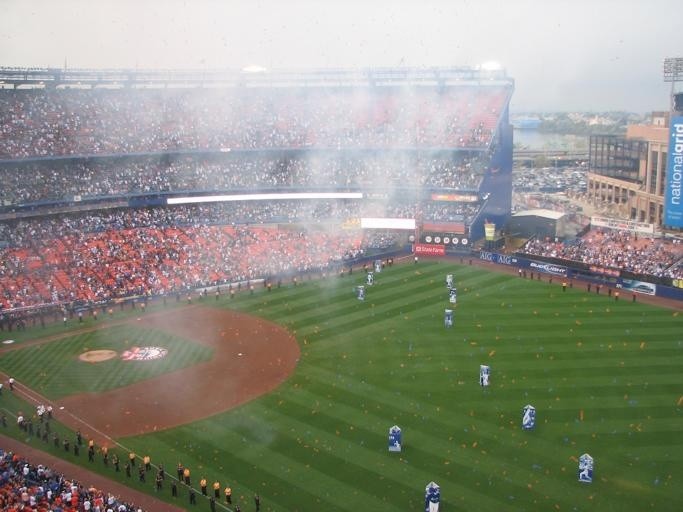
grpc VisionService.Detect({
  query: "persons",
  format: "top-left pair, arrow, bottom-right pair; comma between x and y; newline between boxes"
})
1,63 -> 682,331
478,363 -> 490,387
1,374 -> 262,511
424,481 -> 440,512
388,425 -> 402,451
522,403 -> 535,431
577,452 -> 593,483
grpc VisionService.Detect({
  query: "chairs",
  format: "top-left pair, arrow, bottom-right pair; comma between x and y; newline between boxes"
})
516,220 -> 681,283
0,81 -> 511,319
0,449 -> 137,512
